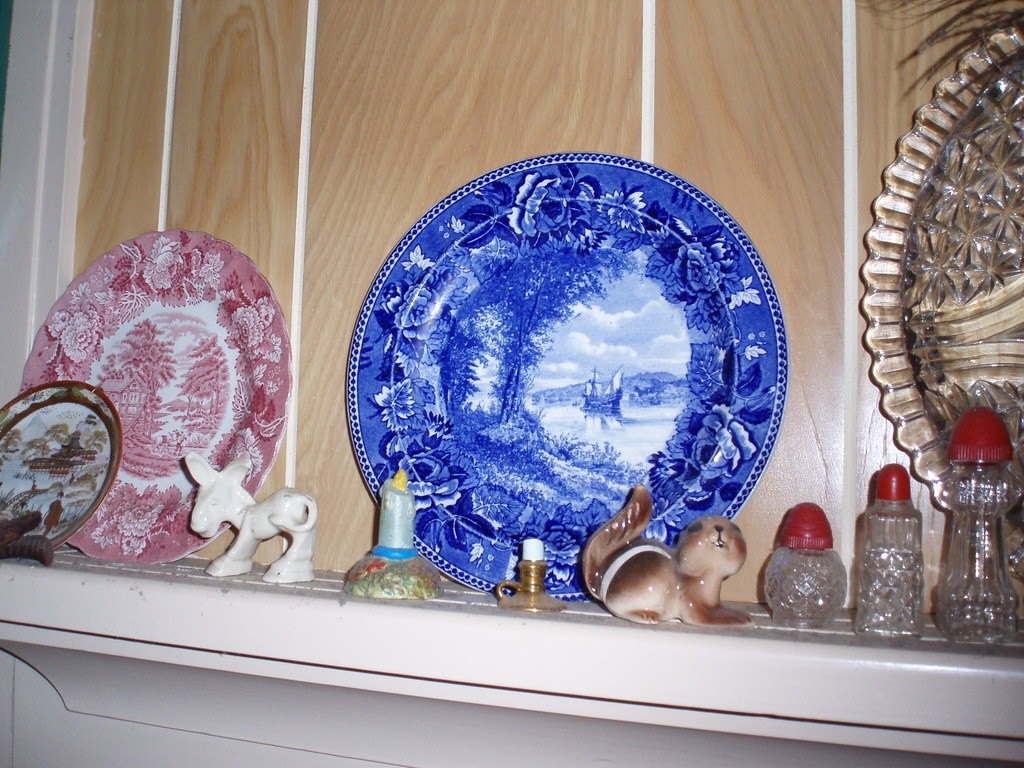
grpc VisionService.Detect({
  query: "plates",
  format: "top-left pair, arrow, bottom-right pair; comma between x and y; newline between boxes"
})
15,229 -> 292,568
0,379 -> 124,559
342,152 -> 789,599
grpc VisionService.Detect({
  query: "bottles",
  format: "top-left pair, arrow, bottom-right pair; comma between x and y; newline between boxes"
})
938,406 -> 1020,648
853,464 -> 925,639
763,502 -> 848,629
